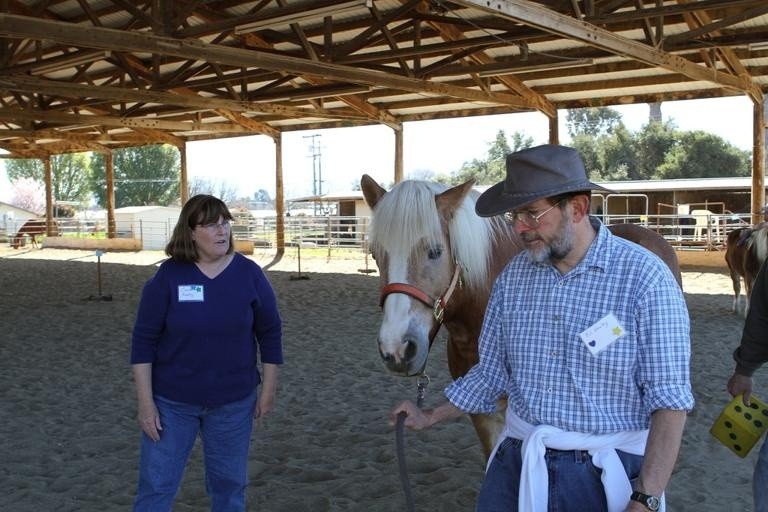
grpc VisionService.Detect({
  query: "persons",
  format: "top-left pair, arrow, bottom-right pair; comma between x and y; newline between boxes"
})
387,143 -> 698,512
724,252 -> 768,512
127,192 -> 285,512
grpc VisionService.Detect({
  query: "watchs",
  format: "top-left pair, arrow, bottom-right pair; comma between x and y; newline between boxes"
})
629,492 -> 662,512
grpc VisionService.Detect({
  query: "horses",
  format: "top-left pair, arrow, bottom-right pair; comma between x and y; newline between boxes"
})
12,218 -> 62,248
357,172 -> 684,462
724,221 -> 768,318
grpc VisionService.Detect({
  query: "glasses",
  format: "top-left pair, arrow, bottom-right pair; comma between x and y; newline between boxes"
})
502,200 -> 561,228
190,220 -> 230,233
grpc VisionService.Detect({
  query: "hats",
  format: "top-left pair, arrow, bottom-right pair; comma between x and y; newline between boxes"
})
474,142 -> 618,217
